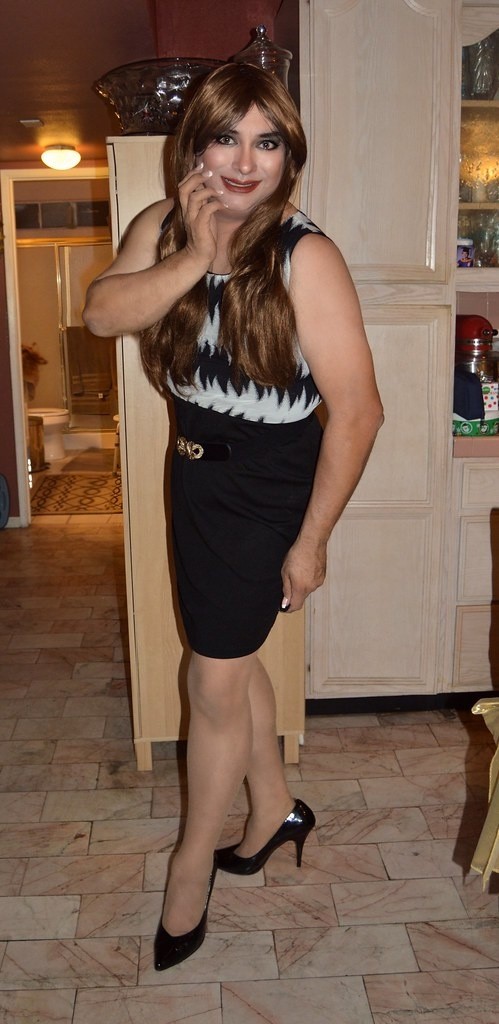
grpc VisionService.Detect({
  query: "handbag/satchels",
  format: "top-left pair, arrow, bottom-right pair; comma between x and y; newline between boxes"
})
454,367 -> 486,420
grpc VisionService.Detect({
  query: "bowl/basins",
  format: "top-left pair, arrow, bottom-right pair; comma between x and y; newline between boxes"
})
94,58 -> 226,136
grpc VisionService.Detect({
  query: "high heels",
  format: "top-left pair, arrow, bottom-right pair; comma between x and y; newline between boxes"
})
153,849 -> 217,972
217,799 -> 316,875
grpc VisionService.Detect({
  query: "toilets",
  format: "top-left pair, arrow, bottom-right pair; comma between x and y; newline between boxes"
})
27,407 -> 71,462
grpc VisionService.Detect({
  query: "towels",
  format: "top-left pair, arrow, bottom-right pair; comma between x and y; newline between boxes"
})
66,326 -> 118,415
22,344 -> 48,401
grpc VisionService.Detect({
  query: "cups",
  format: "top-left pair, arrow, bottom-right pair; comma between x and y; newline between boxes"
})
458,128 -> 473,202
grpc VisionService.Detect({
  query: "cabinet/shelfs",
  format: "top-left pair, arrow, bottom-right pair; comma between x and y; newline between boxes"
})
294,0 -> 499,716
106,134 -> 309,766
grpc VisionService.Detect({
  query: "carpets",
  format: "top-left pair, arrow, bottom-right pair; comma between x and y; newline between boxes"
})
29,474 -> 123,513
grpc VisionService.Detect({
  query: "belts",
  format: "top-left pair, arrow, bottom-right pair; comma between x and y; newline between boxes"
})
176,410 -> 314,462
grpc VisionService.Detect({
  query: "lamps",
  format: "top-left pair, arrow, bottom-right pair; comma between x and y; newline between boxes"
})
40,143 -> 81,171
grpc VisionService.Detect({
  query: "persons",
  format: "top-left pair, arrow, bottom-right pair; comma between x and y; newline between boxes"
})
81,60 -> 385,972
